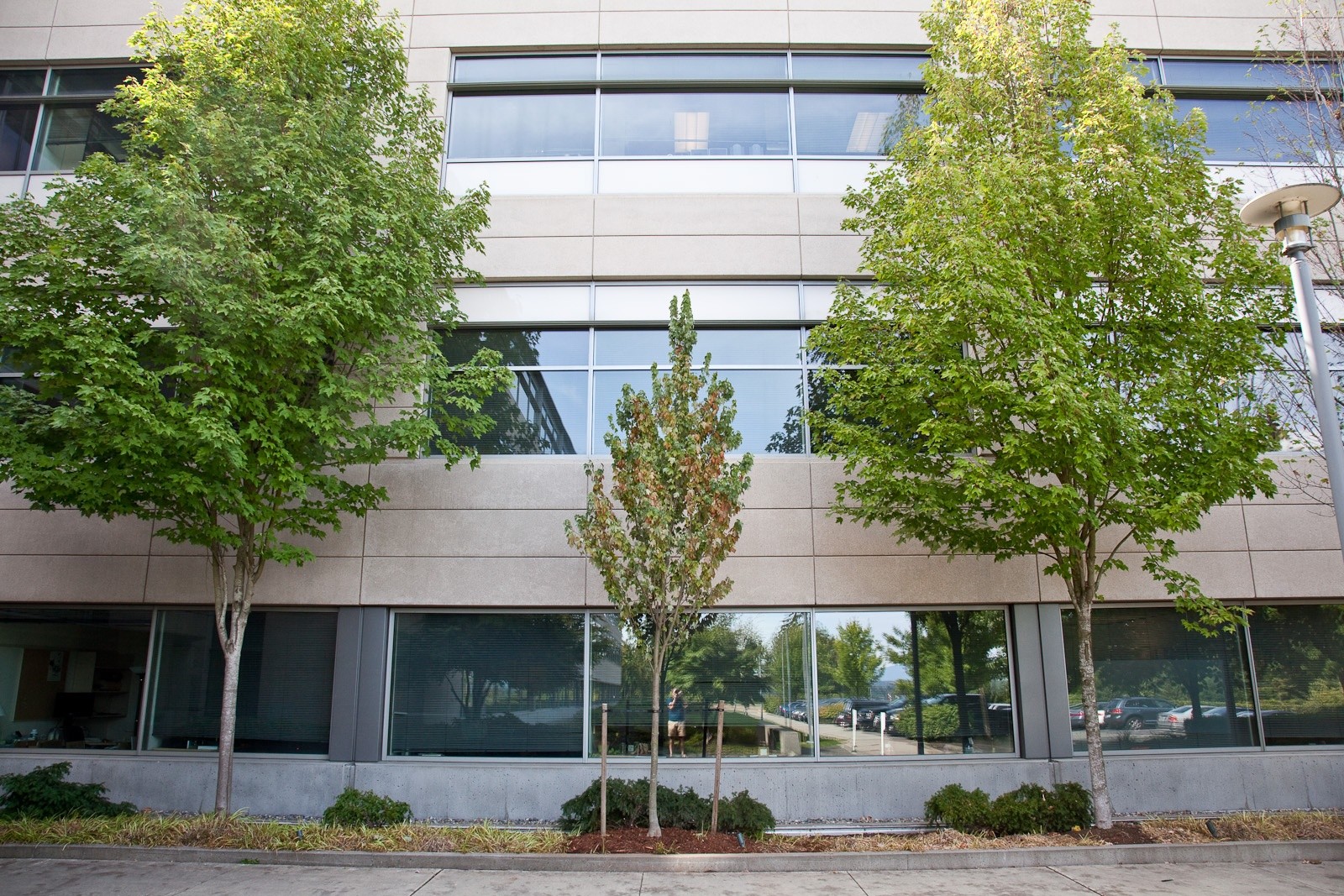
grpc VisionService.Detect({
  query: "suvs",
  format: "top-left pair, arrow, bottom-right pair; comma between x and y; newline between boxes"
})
873,693 -> 983,738
1106,697 -> 1179,729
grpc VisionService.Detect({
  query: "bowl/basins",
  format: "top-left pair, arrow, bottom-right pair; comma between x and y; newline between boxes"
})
598,746 -> 602,752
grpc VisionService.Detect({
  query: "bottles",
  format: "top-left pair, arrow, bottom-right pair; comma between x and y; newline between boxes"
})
621,743 -> 626,754
993,747 -> 995,753
965,743 -> 973,754
988,738 -> 993,753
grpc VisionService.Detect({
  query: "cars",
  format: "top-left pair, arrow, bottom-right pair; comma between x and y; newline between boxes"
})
836,698 -> 891,728
856,698 -> 910,732
1202,706 -> 1291,718
779,695 -> 844,723
1068,701 -> 1087,727
988,703 -> 1012,711
1158,704 -> 1215,732
1097,704 -> 1106,727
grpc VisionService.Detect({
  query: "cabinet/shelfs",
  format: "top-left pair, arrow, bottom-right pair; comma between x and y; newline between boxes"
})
75,666 -> 128,745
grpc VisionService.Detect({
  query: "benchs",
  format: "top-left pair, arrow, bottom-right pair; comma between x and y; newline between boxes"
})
765,724 -> 801,756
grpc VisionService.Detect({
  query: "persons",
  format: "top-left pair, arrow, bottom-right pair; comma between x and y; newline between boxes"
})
665,684 -> 689,758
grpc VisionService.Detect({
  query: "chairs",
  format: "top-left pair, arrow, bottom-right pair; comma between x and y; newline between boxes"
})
691,144 -> 764,155
